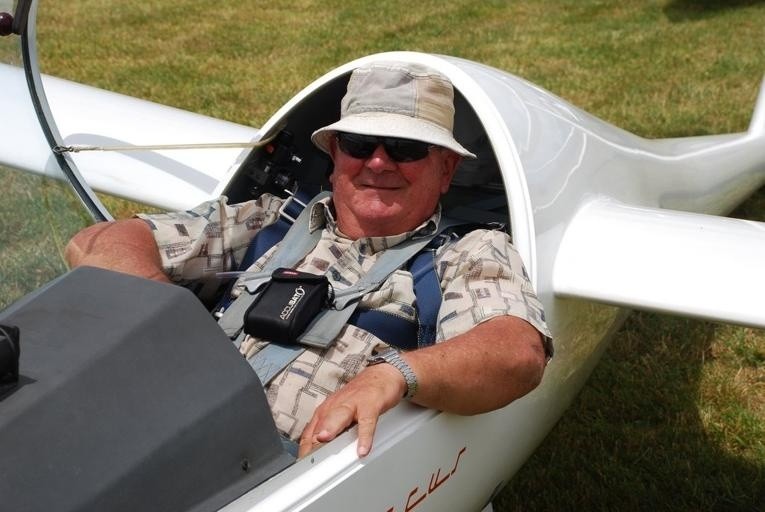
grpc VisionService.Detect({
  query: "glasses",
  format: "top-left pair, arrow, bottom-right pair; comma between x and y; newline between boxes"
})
334,131 -> 436,162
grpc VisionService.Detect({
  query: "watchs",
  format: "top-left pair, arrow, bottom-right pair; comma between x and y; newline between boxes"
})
366,348 -> 418,401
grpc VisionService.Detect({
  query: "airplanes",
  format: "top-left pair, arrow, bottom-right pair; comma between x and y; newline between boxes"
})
0,0 -> 765,512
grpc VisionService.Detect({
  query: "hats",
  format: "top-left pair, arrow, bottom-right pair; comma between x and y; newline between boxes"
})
311,61 -> 478,160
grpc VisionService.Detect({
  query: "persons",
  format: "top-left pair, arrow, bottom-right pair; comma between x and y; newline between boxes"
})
60,60 -> 557,461
